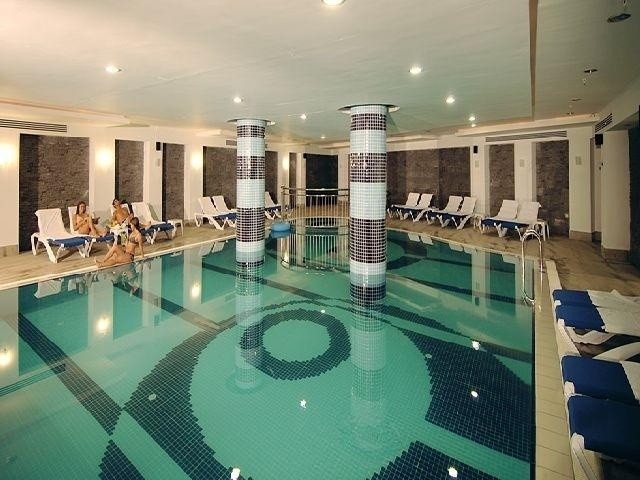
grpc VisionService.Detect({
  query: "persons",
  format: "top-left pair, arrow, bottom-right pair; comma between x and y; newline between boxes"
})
125,262 -> 143,298
71,198 -> 151,269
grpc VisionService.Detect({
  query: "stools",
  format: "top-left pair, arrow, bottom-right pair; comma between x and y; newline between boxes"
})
167,219 -> 183,239
531,220 -> 550,241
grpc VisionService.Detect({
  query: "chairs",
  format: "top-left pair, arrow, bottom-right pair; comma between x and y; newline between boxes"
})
265,191 -> 290,220
501,254 -> 519,264
194,196 -> 238,229
464,247 -> 476,254
409,233 -> 419,242
553,289 -> 640,464
422,237 -> 434,246
67,272 -> 94,291
34,281 -> 62,298
449,243 -> 463,251
200,241 -> 225,255
30,202 -> 172,264
390,192 -> 540,241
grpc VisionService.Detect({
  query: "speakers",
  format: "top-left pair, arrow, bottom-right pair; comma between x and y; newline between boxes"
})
595,134 -> 603,145
474,146 -> 478,153
156,142 -> 160,150
